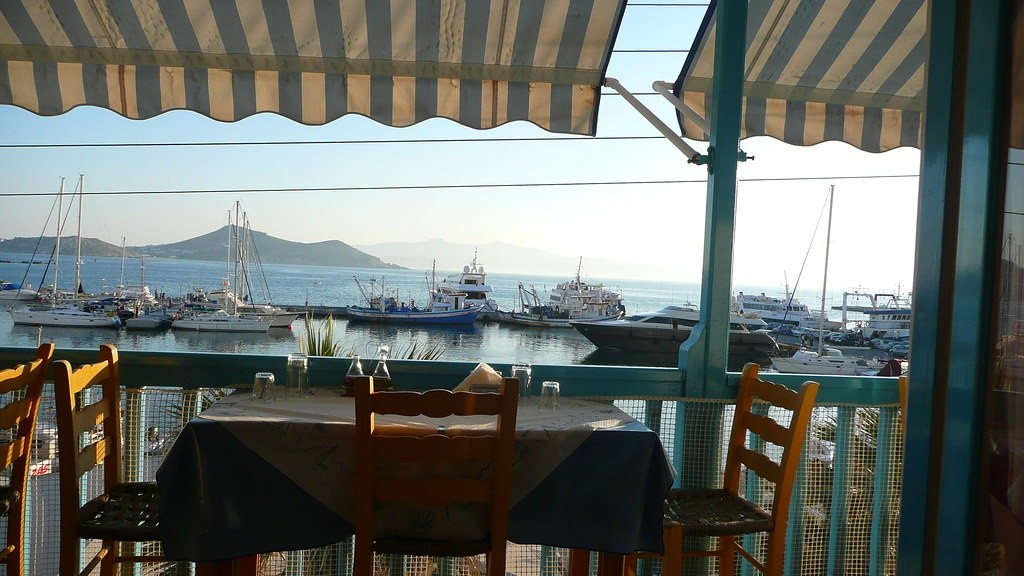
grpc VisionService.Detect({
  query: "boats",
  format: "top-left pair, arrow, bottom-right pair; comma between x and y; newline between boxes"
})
170,310 -> 277,333
429,245 -> 510,322
345,259 -> 486,324
853,282 -> 912,340
730,269 -> 842,331
569,302 -> 780,355
0,279 -> 40,300
495,254 -> 625,328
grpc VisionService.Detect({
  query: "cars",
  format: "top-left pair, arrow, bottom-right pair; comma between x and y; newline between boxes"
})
830,333 -> 842,342
825,332 -> 838,341
793,326 -> 813,337
772,324 -> 793,334
761,322 -> 781,330
791,327 -> 806,335
836,333 -> 853,342
799,328 -> 825,342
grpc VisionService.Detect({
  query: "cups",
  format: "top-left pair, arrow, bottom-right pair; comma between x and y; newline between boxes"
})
253,372 -> 276,404
539,382 -> 561,410
286,354 -> 310,399
512,366 -> 531,408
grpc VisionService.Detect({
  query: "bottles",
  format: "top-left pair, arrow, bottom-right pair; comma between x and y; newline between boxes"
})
346,355 -> 364,395
373,345 -> 393,392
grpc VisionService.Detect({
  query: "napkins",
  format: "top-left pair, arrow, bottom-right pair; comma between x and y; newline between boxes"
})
451,362 -> 502,392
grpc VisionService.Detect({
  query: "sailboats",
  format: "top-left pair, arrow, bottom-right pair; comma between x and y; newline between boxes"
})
176,201 -> 309,328
26,173 -> 142,324
6,178 -> 121,328
766,184 -> 889,374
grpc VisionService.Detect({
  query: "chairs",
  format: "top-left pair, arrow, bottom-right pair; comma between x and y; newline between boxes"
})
353,375 -> 520,575
0,342 -> 57,576
624,363 -> 820,576
53,343 -> 232,576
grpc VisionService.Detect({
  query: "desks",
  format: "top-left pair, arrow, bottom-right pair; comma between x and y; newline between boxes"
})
154,386 -> 673,576
